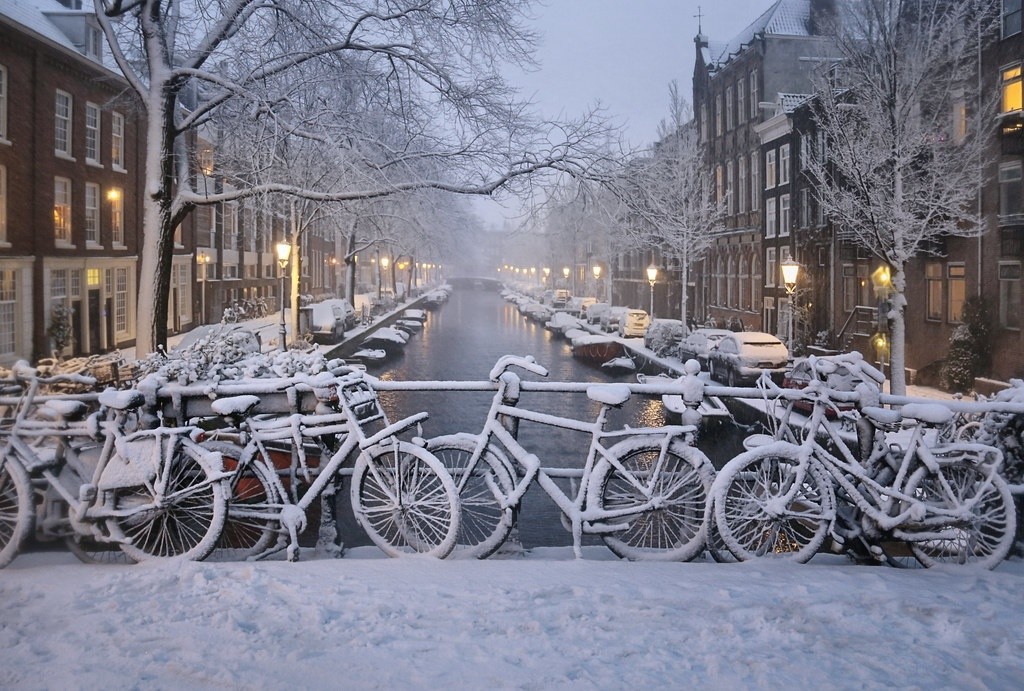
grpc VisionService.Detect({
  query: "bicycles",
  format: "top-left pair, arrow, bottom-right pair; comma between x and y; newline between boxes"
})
0,358 -> 461,564
224,298 -> 269,324
389,356 -> 719,562
707,356 -> 1024,574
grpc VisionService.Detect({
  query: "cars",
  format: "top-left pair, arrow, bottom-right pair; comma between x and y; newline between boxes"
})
677,329 -> 735,372
779,355 -> 886,420
707,332 -> 792,391
644,319 -> 691,358
500,276 -> 633,335
617,309 -> 650,339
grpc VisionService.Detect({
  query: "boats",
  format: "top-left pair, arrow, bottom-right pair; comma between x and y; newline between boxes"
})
498,287 -> 592,344
572,341 -> 623,365
350,279 -> 452,363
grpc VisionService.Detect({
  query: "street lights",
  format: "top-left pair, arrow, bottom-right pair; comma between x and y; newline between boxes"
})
381,255 -> 390,303
275,238 -> 292,353
592,264 -> 601,303
781,254 -> 800,358
647,262 -> 658,326
563,266 -> 570,310
